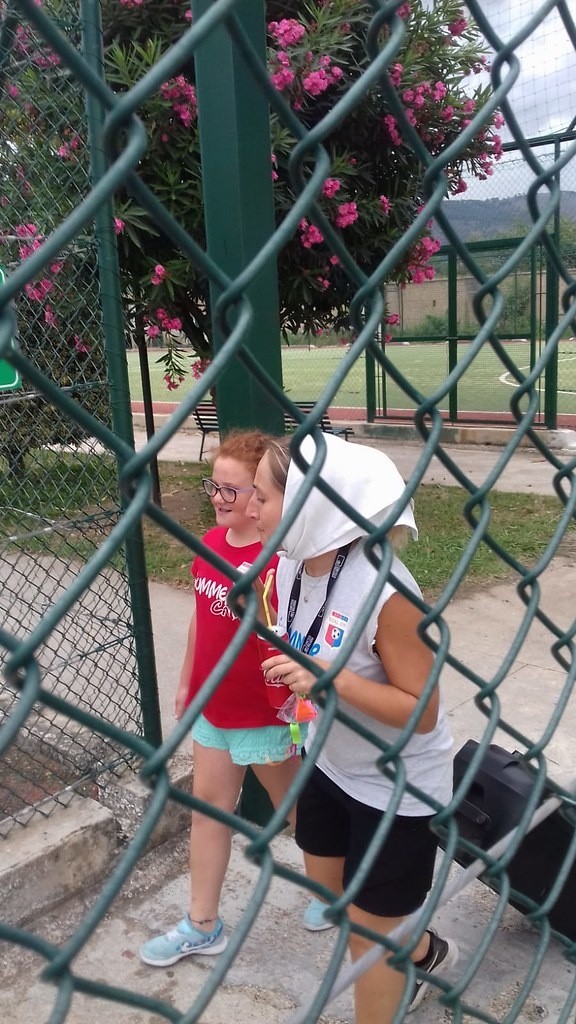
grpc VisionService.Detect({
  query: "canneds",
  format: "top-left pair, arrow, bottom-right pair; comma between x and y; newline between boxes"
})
257,625 -> 296,709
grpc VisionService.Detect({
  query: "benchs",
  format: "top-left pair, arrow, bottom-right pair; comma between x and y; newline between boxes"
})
192,401 -> 356,461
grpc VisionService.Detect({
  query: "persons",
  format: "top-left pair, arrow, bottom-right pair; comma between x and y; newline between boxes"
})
245,433 -> 460,1024
138,432 -> 345,966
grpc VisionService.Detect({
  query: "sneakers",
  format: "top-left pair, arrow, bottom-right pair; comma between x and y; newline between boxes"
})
302,896 -> 334,931
406,928 -> 459,1013
138,913 -> 228,967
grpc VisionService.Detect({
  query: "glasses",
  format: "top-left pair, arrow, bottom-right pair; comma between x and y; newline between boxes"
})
202,477 -> 253,504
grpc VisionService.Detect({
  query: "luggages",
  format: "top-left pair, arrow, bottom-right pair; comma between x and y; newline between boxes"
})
435,740 -> 576,940
